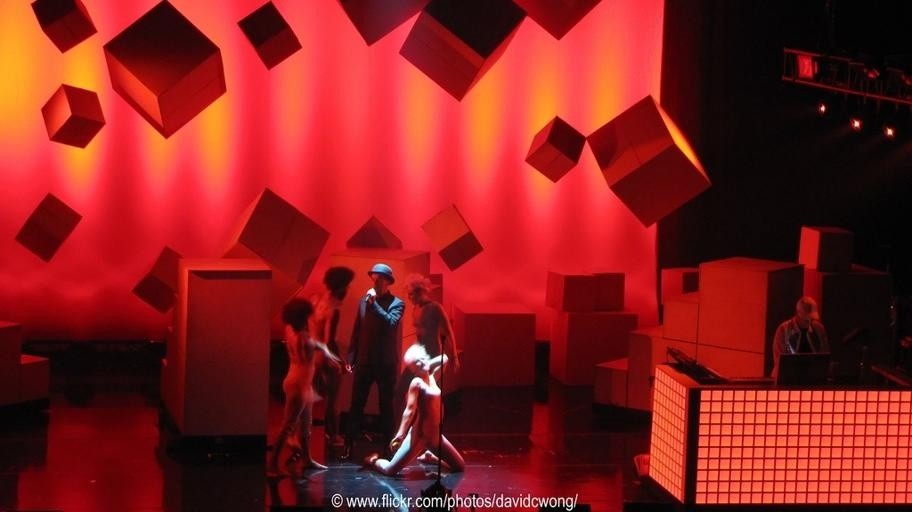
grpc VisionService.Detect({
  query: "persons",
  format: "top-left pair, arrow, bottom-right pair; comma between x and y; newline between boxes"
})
261,297 -> 342,477
361,342 -> 464,473
405,272 -> 461,419
770,296 -> 832,382
310,265 -> 355,450
340,263 -> 405,461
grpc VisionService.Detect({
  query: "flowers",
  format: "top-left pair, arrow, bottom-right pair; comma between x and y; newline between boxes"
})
365,294 -> 371,302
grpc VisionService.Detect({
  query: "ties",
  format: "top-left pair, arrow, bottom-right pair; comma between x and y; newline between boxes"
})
775,351 -> 832,387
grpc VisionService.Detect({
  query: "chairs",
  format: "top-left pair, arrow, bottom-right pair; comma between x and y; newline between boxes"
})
286,449 -> 301,466
357,452 -> 379,467
416,450 -> 432,462
304,460 -> 328,469
341,435 -> 361,461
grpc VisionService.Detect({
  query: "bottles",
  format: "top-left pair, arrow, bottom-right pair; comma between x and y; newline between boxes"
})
369,263 -> 395,284
